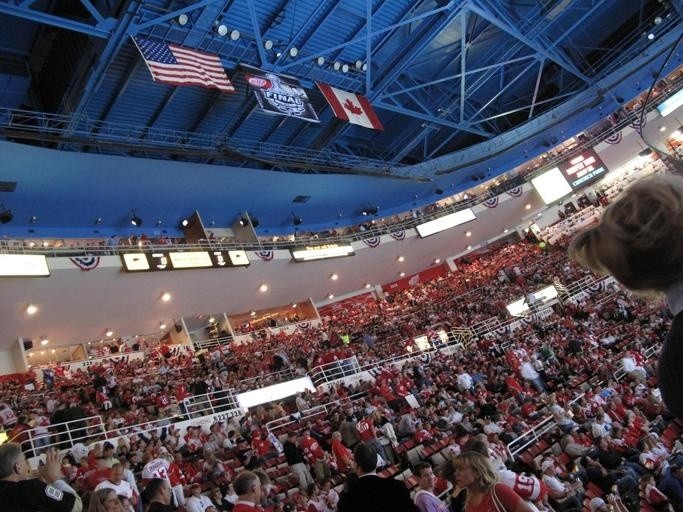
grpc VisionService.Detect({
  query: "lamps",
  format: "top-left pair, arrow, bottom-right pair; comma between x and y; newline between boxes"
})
643,7 -> 672,40
172,14 -> 369,75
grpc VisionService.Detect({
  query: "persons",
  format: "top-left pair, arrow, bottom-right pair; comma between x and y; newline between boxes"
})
569,170 -> 683,432
0,232 -> 250,257
383,191 -> 606,321
263,222 -> 385,250
488,72 -> 683,197
0,297 -> 683,511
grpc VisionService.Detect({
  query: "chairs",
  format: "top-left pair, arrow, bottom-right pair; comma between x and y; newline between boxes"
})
221,336 -> 677,503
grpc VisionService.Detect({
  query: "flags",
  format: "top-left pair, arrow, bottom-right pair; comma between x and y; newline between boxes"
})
129,36 -> 237,93
246,74 -> 321,123
315,81 -> 384,130
